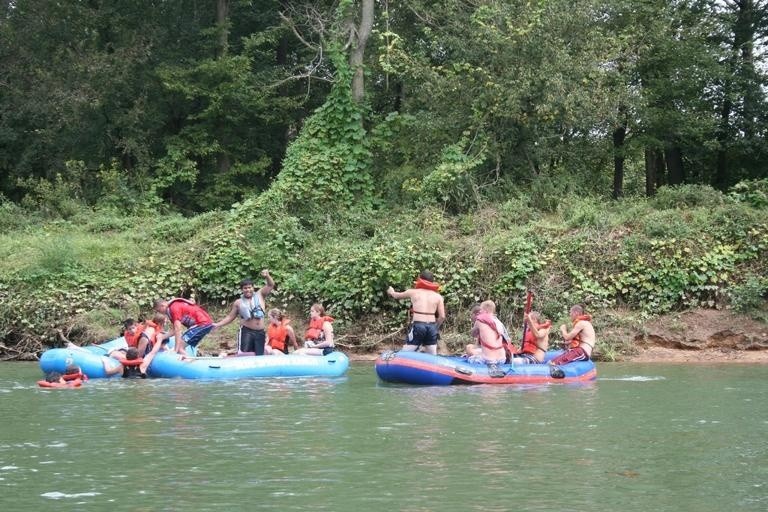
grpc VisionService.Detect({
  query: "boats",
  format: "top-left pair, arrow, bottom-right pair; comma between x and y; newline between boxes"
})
376,351 -> 597,384
39,332 -> 197,379
148,350 -> 348,380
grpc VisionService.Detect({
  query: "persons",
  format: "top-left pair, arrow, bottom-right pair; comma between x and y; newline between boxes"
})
302,301 -> 334,357
465,306 -> 513,363
143,312 -> 175,353
45,368 -> 79,385
512,311 -> 551,366
386,269 -> 445,356
100,332 -> 164,381
211,269 -> 275,356
464,299 -> 508,365
264,306 -> 298,355
546,303 -> 595,366
151,296 -> 213,359
123,317 -> 153,377
